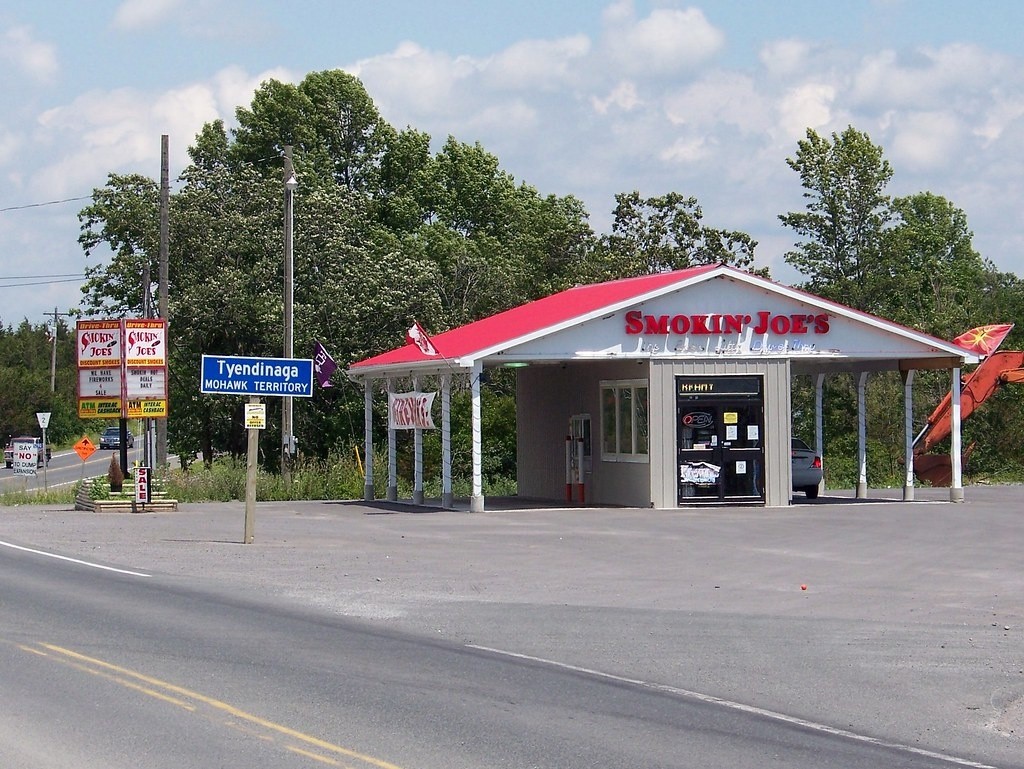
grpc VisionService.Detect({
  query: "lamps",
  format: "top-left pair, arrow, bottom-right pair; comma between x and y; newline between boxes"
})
285,177 -> 298,190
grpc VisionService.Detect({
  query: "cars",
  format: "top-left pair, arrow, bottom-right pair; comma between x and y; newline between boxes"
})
99,427 -> 135,450
791,436 -> 822,499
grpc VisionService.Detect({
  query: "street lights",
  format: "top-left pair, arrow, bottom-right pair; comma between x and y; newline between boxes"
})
281,144 -> 299,485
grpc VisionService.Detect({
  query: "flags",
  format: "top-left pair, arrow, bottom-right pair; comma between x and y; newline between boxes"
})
953,324 -> 1012,355
313,343 -> 337,387
406,322 -> 439,356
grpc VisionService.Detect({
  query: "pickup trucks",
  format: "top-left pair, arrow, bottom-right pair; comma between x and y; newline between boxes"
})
4,436 -> 52,469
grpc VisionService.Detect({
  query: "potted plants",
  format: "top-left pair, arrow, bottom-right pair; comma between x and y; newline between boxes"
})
108,452 -> 124,492
